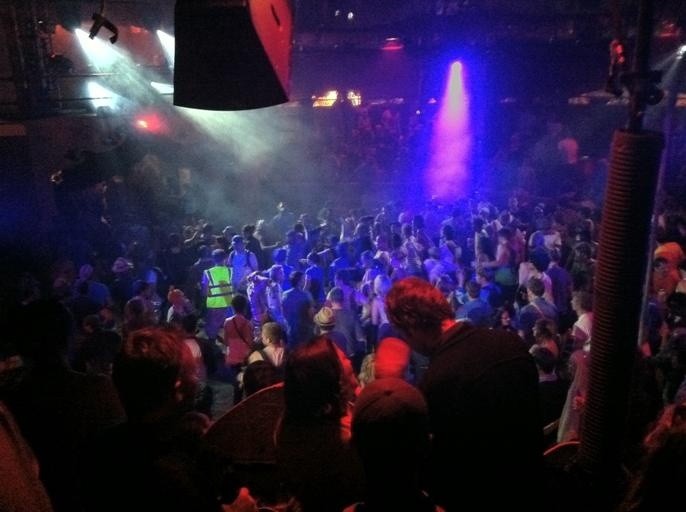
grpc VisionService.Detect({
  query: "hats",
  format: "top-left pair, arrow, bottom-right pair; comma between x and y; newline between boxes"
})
312,306 -> 337,327
351,377 -> 431,501
111,257 -> 134,273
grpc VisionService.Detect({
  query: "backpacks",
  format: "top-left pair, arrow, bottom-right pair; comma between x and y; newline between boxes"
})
182,336 -> 214,366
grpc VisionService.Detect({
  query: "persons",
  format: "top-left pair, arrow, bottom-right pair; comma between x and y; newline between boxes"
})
1,104 -> 685,510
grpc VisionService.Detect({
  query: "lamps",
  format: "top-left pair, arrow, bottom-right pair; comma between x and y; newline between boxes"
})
88,1 -> 117,42
